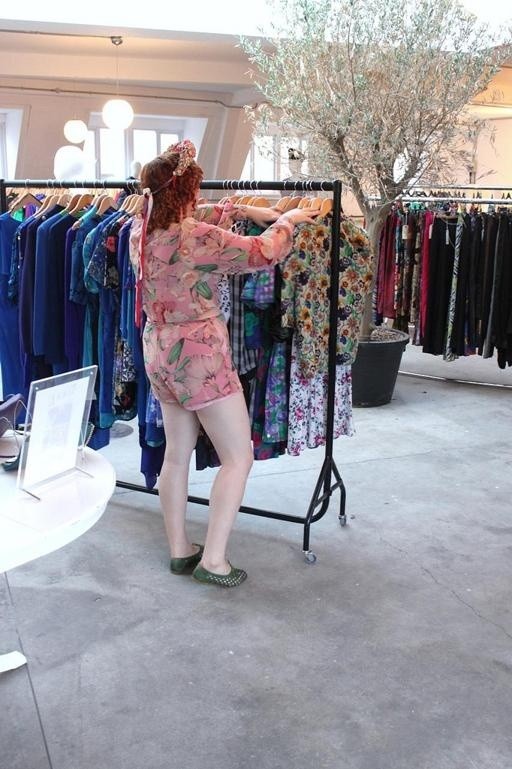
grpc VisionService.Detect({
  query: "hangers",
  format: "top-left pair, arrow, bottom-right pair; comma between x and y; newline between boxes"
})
365,182 -> 512,222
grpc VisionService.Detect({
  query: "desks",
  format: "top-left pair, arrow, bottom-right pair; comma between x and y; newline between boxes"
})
0,430 -> 115,676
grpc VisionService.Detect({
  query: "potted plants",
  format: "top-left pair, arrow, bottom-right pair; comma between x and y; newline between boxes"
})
234,0 -> 512,407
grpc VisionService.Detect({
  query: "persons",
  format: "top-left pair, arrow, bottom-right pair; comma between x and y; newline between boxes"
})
128,137 -> 320,587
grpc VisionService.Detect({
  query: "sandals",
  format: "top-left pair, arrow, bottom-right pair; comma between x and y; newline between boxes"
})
170,543 -> 205,575
192,559 -> 247,588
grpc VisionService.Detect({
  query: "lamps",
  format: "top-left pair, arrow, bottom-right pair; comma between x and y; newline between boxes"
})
63,35 -> 88,145
102,37 -> 134,131
